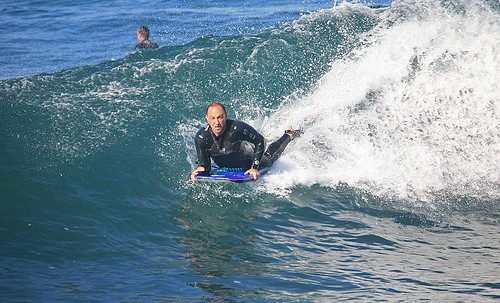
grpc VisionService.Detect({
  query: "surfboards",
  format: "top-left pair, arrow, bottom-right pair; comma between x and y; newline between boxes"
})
195,167 -> 268,182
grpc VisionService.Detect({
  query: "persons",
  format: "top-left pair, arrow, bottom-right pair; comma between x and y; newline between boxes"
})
191,101 -> 305,181
132,25 -> 158,51
